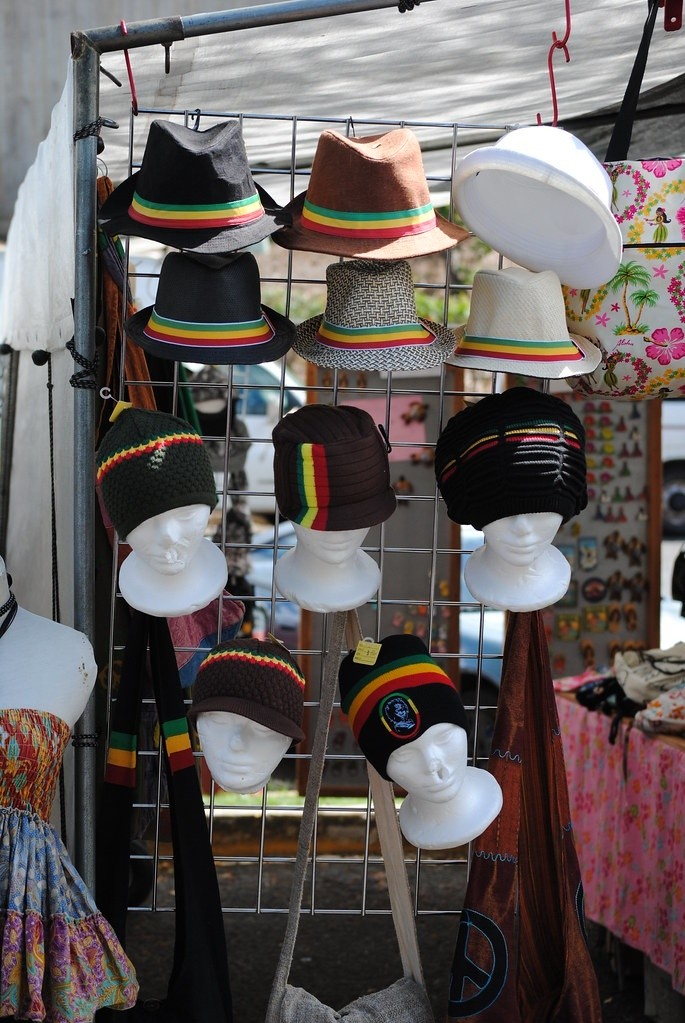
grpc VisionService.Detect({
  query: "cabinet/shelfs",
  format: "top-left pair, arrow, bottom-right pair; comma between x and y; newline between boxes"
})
97,96 -> 574,932
494,158 -> 684,670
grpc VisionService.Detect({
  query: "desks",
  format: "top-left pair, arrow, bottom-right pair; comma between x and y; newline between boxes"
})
538,673 -> 684,1023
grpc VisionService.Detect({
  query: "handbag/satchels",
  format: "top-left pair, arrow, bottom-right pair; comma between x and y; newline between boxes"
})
265,984 -> 428,1022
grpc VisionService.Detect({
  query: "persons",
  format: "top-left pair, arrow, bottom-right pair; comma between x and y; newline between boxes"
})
0,558 -> 140,1023
91,384 -> 587,851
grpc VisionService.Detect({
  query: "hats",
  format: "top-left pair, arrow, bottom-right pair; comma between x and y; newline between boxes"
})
442,269 -> 602,378
105,120 -> 294,254
336,633 -> 469,781
453,125 -> 624,291
272,405 -> 398,534
121,253 -> 295,366
435,387 -> 590,530
272,127 -> 473,261
293,259 -> 455,373
94,408 -> 220,541
189,638 -> 305,745
